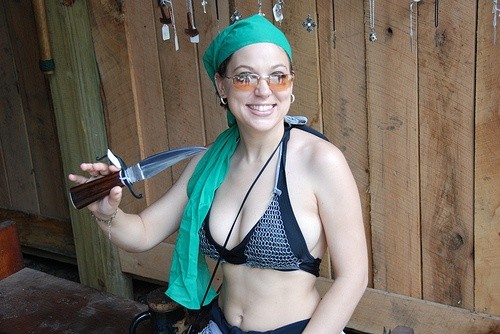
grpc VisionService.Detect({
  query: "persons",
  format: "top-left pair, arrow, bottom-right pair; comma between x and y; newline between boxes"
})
67,14 -> 370,334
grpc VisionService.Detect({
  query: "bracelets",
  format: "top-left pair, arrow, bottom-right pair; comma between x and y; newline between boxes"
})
90,208 -> 118,240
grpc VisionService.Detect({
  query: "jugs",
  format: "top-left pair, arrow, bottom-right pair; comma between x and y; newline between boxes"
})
130,287 -> 197,334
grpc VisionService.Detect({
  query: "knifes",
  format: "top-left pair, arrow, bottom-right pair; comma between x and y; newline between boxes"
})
69,146 -> 207,210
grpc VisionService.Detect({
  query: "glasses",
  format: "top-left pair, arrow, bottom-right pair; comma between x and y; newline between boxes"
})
221,72 -> 295,89
